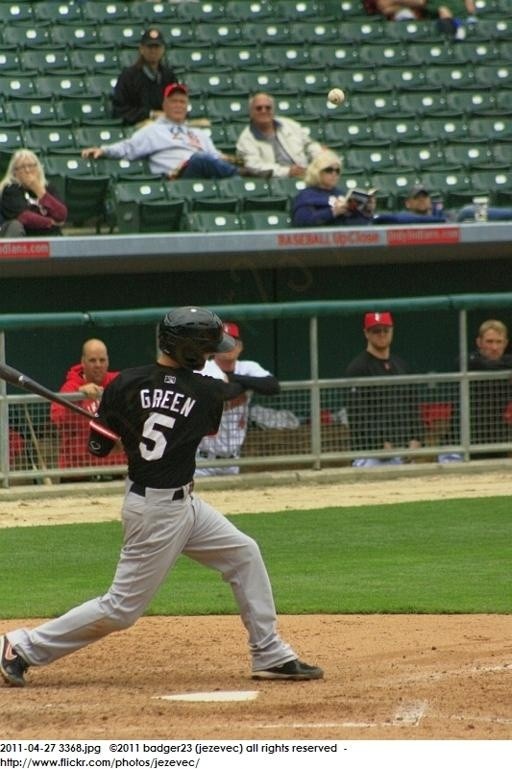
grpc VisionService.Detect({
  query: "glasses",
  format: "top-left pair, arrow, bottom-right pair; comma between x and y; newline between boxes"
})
319,166 -> 343,176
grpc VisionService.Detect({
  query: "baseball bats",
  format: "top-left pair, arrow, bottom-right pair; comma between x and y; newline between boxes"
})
14,404 -> 43,485
0,363 -> 94,419
23,404 -> 52,484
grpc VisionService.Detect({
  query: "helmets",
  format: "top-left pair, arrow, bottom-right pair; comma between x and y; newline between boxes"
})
155,304 -> 225,371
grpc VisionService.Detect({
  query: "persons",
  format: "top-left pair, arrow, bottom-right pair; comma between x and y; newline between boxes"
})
192,324 -> 279,481
436,320 -> 512,458
0,147 -> 67,237
236,95 -> 329,176
110,27 -> 178,125
404,189 -> 448,218
363,0 -> 479,32
83,84 -> 273,178
50,338 -> 137,482
290,149 -> 377,228
332,312 -> 422,465
1,307 -> 324,686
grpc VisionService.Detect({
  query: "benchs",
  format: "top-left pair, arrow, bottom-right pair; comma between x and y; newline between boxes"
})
242,409 -> 451,471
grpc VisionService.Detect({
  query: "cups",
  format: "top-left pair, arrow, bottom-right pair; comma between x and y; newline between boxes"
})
471,196 -> 489,221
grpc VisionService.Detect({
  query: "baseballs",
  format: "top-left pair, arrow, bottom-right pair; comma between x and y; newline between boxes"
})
328,88 -> 346,105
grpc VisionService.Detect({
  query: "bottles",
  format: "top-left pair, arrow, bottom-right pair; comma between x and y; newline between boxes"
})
465,12 -> 479,37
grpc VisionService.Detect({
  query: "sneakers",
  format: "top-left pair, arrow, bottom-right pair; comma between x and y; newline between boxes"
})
1,633 -> 29,687
249,655 -> 323,681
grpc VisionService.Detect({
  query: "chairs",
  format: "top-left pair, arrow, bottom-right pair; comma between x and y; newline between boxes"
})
0,0 -> 510,232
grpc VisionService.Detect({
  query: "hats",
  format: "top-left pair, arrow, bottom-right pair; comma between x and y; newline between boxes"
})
222,320 -> 242,339
408,182 -> 431,195
138,27 -> 167,46
363,310 -> 395,328
162,81 -> 187,95
